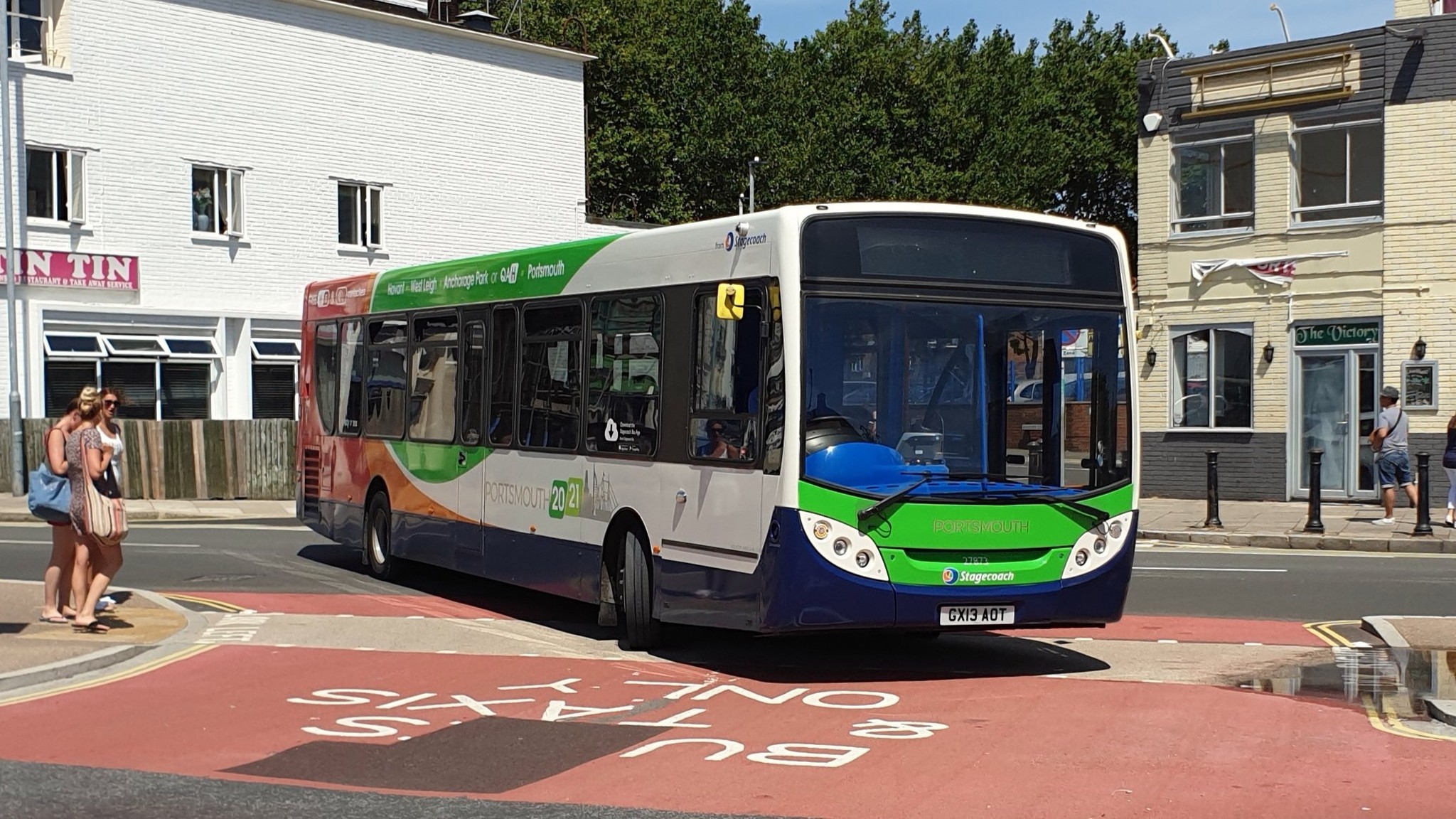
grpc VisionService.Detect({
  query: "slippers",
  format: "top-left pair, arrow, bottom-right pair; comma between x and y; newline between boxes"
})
71,618 -> 109,634
39,609 -> 76,624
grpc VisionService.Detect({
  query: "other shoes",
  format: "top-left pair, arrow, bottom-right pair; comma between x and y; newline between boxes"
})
95,595 -> 116,611
1445,513 -> 1456,529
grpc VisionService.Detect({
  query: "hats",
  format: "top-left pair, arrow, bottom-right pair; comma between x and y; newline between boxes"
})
1379,386 -> 1400,399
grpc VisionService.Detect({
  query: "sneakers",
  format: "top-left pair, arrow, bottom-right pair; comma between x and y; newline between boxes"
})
1371,516 -> 1396,526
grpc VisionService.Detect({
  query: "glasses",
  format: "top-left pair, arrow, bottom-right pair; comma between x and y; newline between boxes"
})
28,464 -> 72,524
83,474 -> 129,547
706,426 -> 724,434
104,398 -> 120,407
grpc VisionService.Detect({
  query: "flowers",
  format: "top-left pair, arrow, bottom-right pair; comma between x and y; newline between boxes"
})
193,188 -> 212,214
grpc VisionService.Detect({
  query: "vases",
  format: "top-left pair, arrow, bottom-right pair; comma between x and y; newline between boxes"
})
198,213 -> 209,231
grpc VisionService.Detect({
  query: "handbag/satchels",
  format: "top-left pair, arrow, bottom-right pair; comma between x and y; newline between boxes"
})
1368,428 -> 1386,451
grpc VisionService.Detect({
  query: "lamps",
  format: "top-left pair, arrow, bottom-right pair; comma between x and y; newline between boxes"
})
1147,348 -> 1157,366
1415,336 -> 1426,359
1263,341 -> 1274,363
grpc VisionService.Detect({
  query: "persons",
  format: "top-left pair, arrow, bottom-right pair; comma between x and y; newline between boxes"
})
65,386 -> 123,633
1372,386 -> 1431,526
87,388 -> 124,610
1442,410 -> 1456,528
40,397 -> 82,623
696,420 -> 738,460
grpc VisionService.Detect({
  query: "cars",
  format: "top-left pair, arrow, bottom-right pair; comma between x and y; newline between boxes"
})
1005,380 -> 1046,401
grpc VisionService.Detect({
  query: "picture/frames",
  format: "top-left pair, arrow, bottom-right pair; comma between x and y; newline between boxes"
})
1401,361 -> 1438,411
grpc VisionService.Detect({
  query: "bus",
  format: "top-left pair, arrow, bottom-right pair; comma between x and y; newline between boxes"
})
292,202 -> 1142,650
